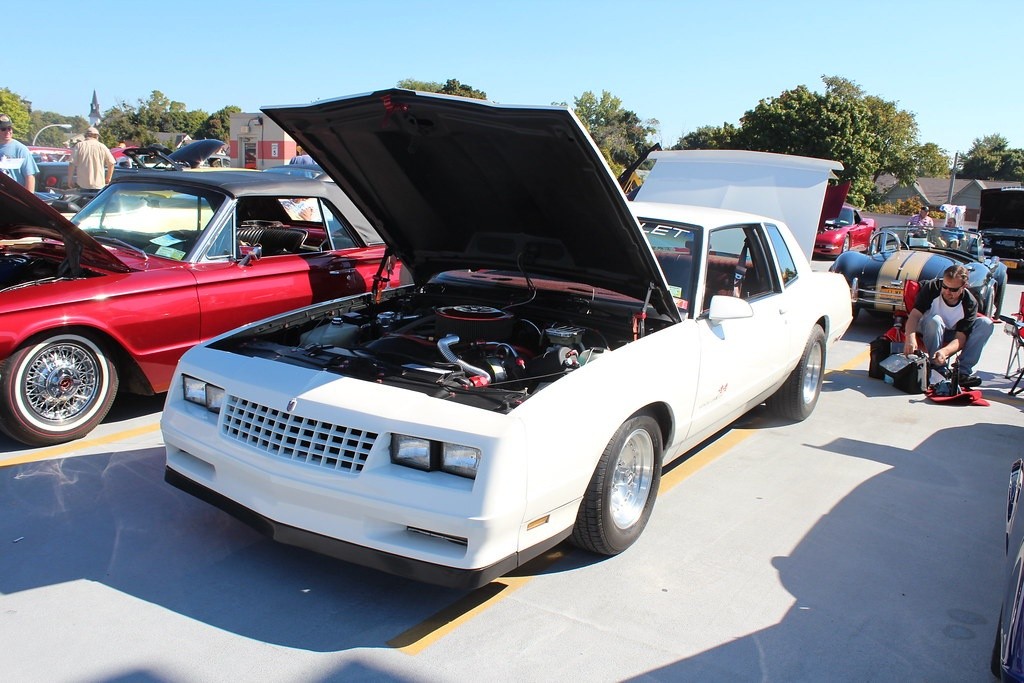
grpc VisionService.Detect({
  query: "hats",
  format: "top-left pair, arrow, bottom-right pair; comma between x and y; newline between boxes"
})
921,207 -> 931,212
0,114 -> 14,128
85,127 -> 100,136
118,143 -> 126,148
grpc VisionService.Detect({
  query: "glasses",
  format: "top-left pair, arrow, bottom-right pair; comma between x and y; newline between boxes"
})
942,283 -> 965,292
0,127 -> 12,131
948,222 -> 953,224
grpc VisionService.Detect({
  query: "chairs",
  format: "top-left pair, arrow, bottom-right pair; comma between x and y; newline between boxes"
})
892,272 -> 1002,396
999,314 -> 1024,396
1004,292 -> 1024,380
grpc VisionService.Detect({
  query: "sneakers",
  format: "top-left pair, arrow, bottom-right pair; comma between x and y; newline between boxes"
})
959,375 -> 982,387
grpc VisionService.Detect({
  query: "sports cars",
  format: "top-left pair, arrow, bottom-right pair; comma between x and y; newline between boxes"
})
830,226 -> 1008,325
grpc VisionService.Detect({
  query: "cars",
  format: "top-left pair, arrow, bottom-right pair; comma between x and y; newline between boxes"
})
968,185 -> 1024,282
813,205 -> 878,259
161,88 -> 854,592
0,171 -> 414,447
35,139 -> 225,193
265,164 -> 335,183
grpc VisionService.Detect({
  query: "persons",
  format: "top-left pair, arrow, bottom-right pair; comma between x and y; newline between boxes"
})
903,265 -> 994,387
0,114 -> 40,194
289,145 -> 318,165
280,198 -> 334,222
67,126 -> 115,193
117,143 -> 132,168
905,206 -> 935,246
936,218 -> 966,250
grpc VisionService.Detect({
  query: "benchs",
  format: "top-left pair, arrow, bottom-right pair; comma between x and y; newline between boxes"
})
281,225 -> 343,251
655,252 -> 754,308
217,229 -> 308,256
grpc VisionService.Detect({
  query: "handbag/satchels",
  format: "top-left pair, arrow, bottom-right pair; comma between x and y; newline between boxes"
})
879,350 -> 927,394
869,335 -> 892,379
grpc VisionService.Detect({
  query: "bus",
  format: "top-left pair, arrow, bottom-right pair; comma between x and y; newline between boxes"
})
27,147 -> 74,162
